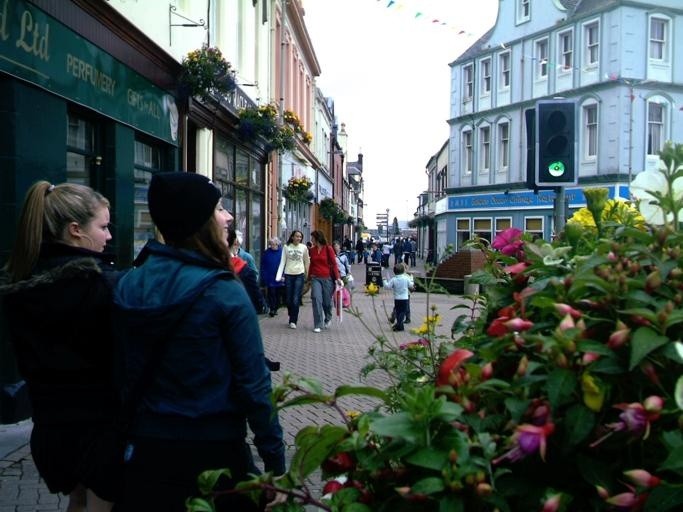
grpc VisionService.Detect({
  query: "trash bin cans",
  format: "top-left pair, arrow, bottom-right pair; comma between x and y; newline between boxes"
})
366,262 -> 383,287
465,275 -> 480,296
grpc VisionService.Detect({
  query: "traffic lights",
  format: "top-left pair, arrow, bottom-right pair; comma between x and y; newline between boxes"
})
524,99 -> 581,191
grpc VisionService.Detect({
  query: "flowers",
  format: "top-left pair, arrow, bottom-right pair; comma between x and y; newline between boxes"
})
179,46 -> 237,97
284,175 -> 314,202
239,102 -> 312,155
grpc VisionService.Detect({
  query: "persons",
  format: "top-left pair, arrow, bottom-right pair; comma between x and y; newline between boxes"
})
228,229 -> 417,333
0,180 -> 127,512
113,171 -> 289,512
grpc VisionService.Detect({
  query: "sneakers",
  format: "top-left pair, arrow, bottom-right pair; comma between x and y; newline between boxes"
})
388,317 -> 410,331
269,310 -> 279,317
325,319 -> 332,329
289,322 -> 297,329
314,327 -> 321,333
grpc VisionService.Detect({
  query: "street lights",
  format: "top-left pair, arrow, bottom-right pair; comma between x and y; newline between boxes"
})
386,208 -> 390,243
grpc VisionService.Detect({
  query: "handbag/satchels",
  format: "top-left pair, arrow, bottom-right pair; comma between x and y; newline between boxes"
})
334,287 -> 350,308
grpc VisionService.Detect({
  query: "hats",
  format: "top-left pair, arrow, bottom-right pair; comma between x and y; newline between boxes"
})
148,171 -> 222,242
